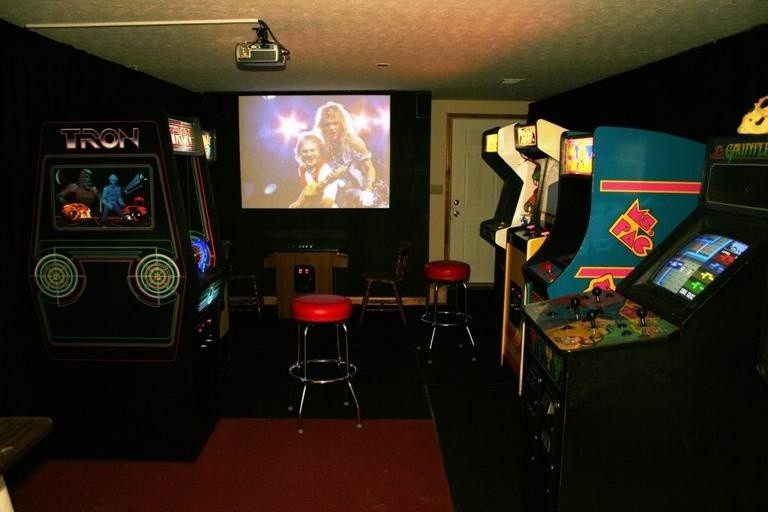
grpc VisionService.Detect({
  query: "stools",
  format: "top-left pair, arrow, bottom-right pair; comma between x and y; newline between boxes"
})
417,260 -> 480,364
287,293 -> 363,434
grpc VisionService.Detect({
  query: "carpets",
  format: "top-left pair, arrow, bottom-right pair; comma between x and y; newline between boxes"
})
4,418 -> 453,511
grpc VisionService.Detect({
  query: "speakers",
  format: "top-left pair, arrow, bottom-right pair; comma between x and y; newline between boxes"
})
415,94 -> 429,120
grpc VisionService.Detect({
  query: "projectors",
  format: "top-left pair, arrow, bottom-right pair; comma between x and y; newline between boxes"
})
234,41 -> 287,71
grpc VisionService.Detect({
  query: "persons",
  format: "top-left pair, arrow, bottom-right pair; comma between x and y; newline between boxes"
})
296,135 -> 339,209
313,102 -> 376,209
56,168 -> 148,226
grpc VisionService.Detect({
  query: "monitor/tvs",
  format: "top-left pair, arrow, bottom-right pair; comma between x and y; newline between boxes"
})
50,164 -> 155,232
650,231 -> 751,302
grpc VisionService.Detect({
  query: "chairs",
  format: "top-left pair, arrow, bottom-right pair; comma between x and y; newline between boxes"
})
216,239 -> 264,320
359,240 -> 410,327
